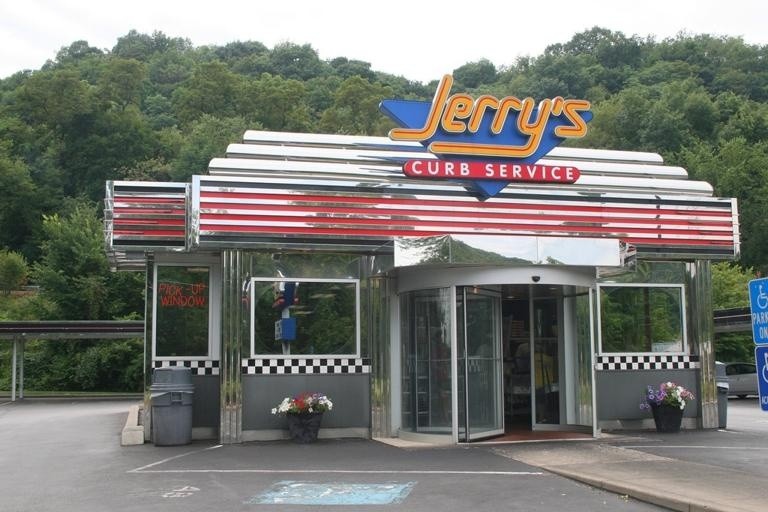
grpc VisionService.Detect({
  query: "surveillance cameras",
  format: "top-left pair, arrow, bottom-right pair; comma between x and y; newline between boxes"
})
532,276 -> 541,282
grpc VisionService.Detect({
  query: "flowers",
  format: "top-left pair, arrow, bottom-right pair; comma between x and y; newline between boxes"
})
270,392 -> 334,418
638,380 -> 698,412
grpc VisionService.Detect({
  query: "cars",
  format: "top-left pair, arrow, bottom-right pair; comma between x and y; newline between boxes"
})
724,361 -> 759,398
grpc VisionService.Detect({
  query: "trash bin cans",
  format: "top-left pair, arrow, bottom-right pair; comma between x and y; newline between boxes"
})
715,360 -> 729,428
149,366 -> 196,446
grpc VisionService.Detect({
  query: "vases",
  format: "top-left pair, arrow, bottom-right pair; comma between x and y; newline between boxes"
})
652,406 -> 682,433
285,412 -> 322,443
